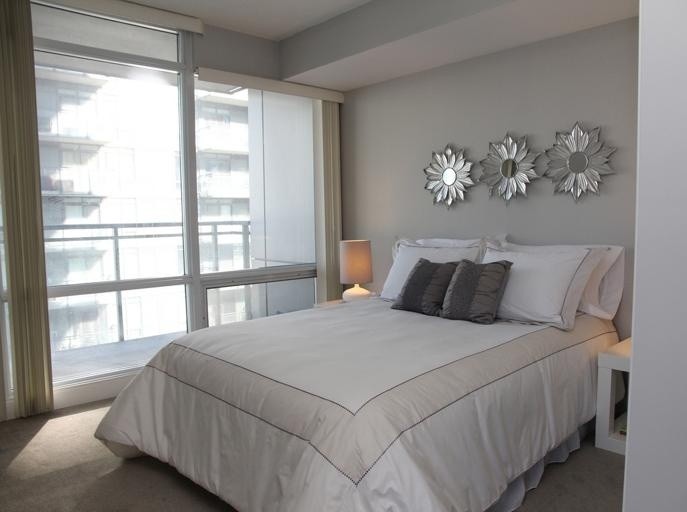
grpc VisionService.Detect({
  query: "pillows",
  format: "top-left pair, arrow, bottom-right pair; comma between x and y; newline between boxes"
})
380,237 -> 625,328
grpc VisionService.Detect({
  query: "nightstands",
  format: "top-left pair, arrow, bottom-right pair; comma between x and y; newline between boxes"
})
594,333 -> 631,455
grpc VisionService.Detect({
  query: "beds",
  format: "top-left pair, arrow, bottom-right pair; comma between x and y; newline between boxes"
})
95,297 -> 611,511
338,238 -> 373,300
